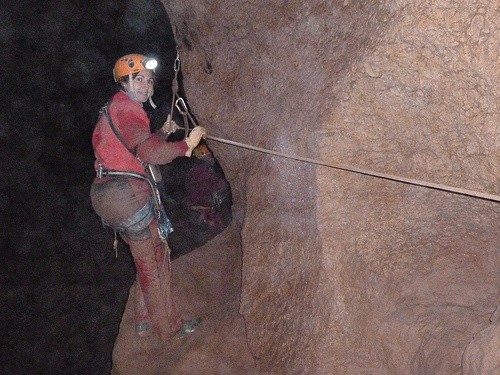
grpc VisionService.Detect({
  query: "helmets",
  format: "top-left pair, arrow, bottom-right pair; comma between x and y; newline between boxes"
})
113,54 -> 156,83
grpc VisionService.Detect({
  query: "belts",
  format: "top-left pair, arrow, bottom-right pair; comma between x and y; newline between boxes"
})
93,169 -> 153,183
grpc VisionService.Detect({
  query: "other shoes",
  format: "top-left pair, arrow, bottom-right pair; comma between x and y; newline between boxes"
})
136,320 -> 157,340
171,315 -> 204,344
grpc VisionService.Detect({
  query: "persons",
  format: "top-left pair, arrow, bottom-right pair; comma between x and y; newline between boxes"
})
91,54 -> 203,345
